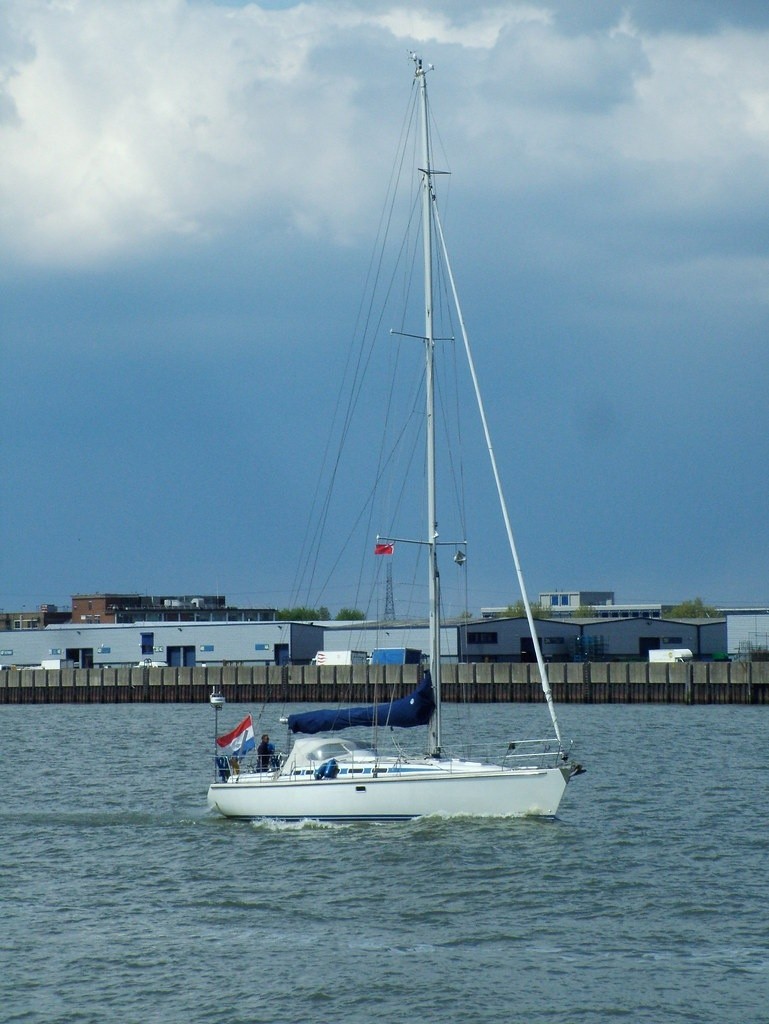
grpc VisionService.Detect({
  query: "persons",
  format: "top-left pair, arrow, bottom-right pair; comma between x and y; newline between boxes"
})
255,734 -> 275,773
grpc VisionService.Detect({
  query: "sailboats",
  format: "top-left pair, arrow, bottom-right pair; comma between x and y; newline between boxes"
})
735,612 -> 769,662
202,64 -> 585,834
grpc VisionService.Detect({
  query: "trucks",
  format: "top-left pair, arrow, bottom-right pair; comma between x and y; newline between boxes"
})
42,658 -> 74,670
366,647 -> 423,665
648,649 -> 694,663
133,661 -> 169,667
309,650 -> 367,665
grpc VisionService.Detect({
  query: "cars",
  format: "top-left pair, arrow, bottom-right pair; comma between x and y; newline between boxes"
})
74,662 -> 79,668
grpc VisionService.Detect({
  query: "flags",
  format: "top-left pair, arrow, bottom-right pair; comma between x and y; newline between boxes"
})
216,715 -> 255,763
374,544 -> 394,554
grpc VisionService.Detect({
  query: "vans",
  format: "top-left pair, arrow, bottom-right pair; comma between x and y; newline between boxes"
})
0,665 -> 11,670
